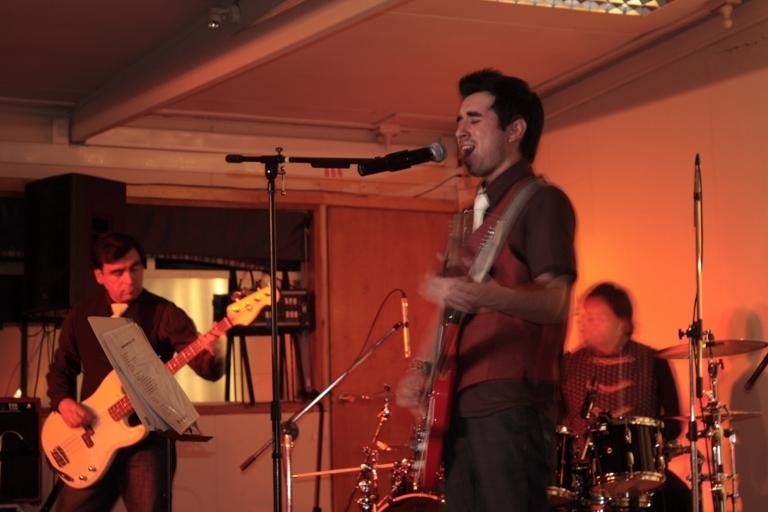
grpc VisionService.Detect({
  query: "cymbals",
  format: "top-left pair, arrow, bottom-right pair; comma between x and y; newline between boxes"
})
655,340 -> 764,359
671,409 -> 758,425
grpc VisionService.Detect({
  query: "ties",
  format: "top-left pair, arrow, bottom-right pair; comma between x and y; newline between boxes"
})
109,302 -> 127,317
470,186 -> 492,235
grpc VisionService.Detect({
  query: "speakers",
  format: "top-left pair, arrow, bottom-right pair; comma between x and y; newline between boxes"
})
21,173 -> 129,313
1,396 -> 46,506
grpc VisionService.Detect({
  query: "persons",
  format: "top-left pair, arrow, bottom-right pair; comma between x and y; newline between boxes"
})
390,65 -> 581,510
564,278 -> 690,510
45,229 -> 225,512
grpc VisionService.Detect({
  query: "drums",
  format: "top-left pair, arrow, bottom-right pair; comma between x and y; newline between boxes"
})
545,425 -> 577,505
584,417 -> 666,497
368,490 -> 445,511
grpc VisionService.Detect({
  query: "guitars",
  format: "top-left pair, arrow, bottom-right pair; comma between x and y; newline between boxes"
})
41,283 -> 280,490
411,220 -> 506,488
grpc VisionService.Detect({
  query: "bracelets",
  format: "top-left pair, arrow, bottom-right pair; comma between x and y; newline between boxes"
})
408,356 -> 433,376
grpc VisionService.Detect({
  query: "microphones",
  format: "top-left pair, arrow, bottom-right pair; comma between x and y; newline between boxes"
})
402,295 -> 412,359
578,388 -> 600,421
357,141 -> 446,177
